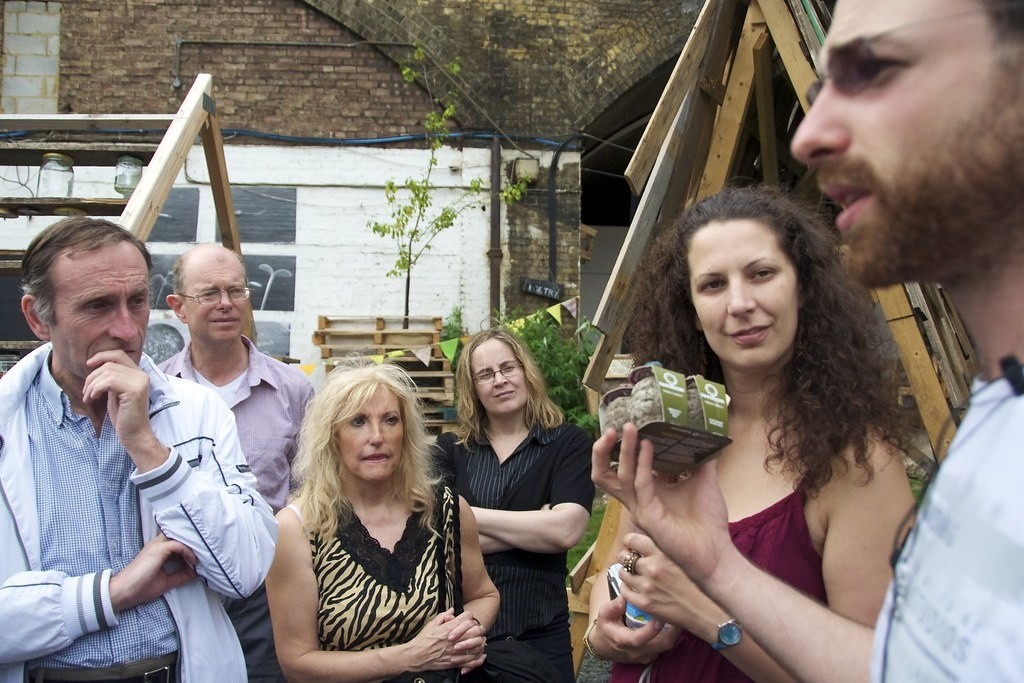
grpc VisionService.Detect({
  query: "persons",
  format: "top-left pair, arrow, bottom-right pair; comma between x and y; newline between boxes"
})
591,0 -> 1024,682
263,352 -> 501,683
434,330 -> 595,683
582,188 -> 914,683
152,246 -> 314,681
0,216 -> 277,683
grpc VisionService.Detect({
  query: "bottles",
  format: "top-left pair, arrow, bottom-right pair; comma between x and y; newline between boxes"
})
36,152 -> 74,197
623,528 -> 687,636
113,153 -> 143,195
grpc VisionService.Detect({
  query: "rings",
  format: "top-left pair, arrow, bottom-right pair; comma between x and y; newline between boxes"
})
622,551 -> 641,574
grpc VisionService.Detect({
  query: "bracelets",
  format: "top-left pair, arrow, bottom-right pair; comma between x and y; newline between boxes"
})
472,616 -> 481,626
583,619 -> 603,660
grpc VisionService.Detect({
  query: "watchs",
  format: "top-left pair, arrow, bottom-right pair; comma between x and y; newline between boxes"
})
712,617 -> 744,650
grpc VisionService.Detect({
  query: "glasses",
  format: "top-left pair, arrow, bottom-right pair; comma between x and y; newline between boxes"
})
178,286 -> 250,304
804,10 -> 993,107
473,363 -> 524,384
